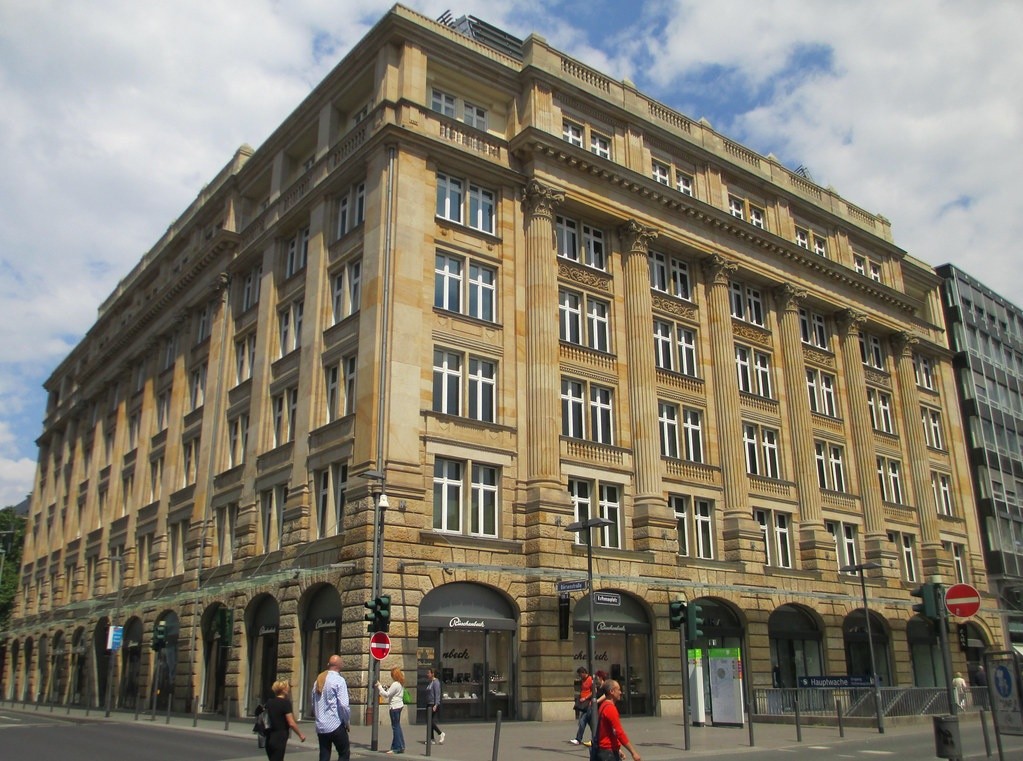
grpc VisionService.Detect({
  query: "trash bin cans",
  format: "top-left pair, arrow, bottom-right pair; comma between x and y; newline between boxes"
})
932,714 -> 962,758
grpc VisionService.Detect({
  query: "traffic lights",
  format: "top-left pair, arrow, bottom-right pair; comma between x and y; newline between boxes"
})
156,625 -> 167,643
909,583 -> 936,619
687,602 -> 704,643
364,595 -> 390,632
669,600 -> 687,630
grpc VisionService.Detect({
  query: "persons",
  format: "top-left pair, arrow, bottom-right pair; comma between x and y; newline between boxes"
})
376,668 -> 406,754
424,667 -> 445,744
311,654 -> 351,761
570,667 -> 641,761
265,679 -> 305,761
973,665 -> 989,711
951,672 -> 966,710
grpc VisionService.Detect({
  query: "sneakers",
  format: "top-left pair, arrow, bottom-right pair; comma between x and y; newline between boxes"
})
439,732 -> 445,743
425,738 -> 436,745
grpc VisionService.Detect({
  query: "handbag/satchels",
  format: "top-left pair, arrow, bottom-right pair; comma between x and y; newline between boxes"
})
253,709 -> 271,737
573,697 -> 590,712
403,687 -> 413,704
590,739 -> 599,761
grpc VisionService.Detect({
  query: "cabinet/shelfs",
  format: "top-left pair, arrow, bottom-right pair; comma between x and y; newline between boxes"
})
574,677 -> 642,698
441,679 -> 508,700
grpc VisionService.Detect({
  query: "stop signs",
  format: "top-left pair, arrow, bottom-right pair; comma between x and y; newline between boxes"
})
370,632 -> 391,660
944,583 -> 981,618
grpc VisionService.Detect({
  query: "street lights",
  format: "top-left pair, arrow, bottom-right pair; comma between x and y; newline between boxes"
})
839,562 -> 886,733
562,517 -> 616,740
356,470 -> 387,752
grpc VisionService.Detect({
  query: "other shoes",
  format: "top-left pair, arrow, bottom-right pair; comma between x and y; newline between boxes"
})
570,739 -> 580,745
387,750 -> 404,754
583,741 -> 592,747
